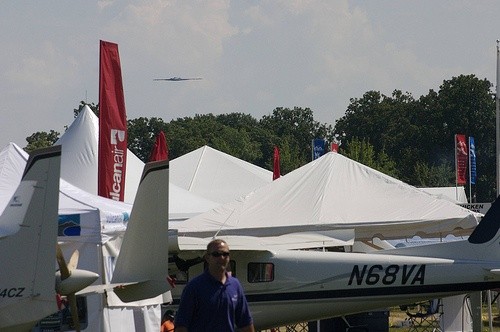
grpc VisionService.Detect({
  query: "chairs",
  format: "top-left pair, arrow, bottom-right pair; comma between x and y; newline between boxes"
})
405,299 -> 443,332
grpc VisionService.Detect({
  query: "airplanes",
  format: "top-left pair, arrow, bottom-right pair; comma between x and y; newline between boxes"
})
103,192 -> 500,332
55,240 -> 99,332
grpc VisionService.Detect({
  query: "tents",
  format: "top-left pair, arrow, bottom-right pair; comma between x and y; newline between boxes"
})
0,104 -> 487,332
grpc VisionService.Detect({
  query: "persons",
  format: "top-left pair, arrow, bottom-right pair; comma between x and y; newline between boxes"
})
172,239 -> 255,332
161,315 -> 174,332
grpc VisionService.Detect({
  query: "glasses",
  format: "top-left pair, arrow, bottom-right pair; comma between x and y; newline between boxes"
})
208,252 -> 230,257
